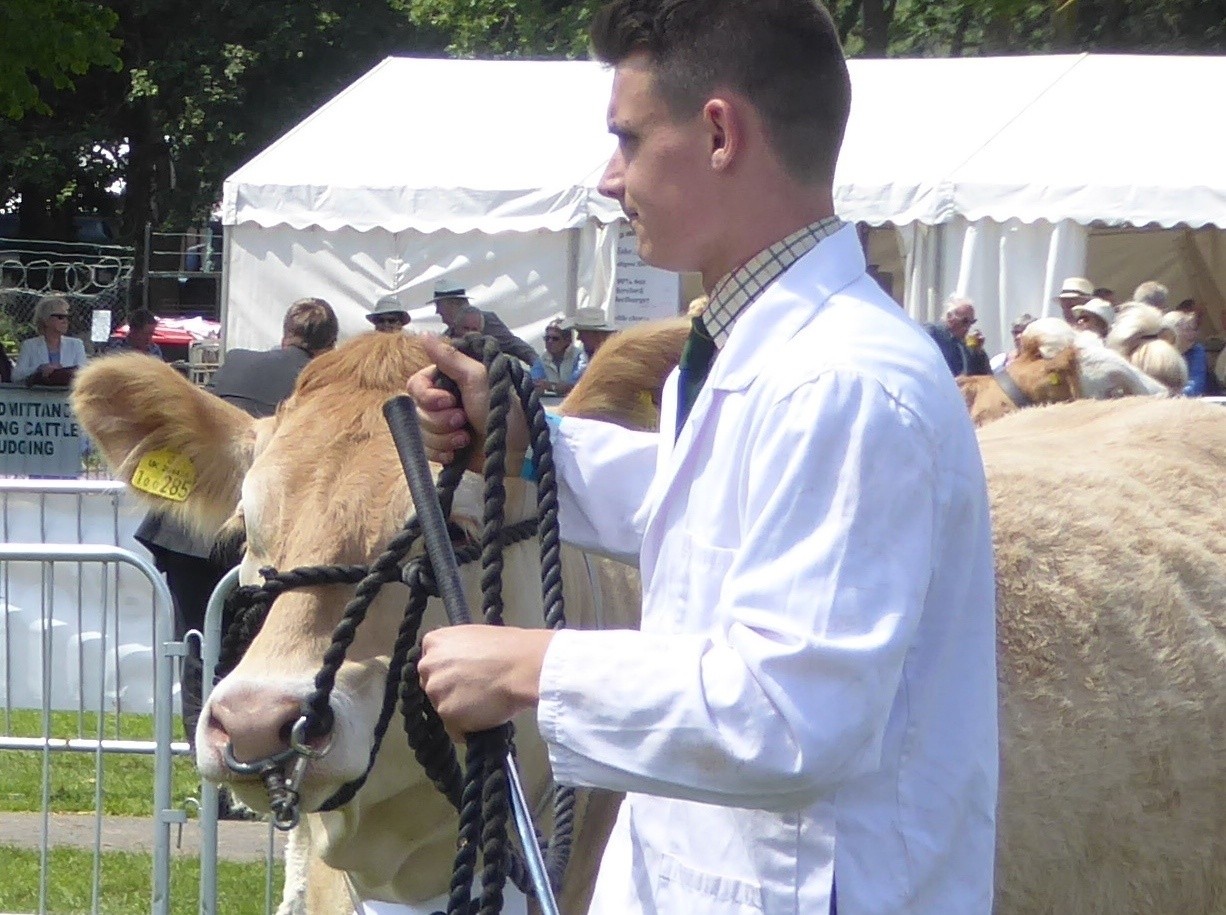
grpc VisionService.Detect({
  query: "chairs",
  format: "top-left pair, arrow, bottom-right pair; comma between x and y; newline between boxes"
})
185,342 -> 222,385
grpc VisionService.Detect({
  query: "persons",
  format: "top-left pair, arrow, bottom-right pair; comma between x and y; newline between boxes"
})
557,307 -> 617,392
530,318 -> 588,396
12,295 -> 87,385
103,309 -> 163,360
1004,277 -> 1207,395
365,295 -> 411,333
920,291 -> 993,378
202,298 -> 339,421
425,278 -> 539,368
406,1 -> 999,915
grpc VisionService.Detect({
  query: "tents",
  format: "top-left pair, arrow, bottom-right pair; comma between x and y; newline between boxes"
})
222,54 -> 1226,361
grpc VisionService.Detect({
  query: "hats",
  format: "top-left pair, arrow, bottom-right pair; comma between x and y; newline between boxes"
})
1072,297 -> 1115,327
425,277 -> 474,304
558,306 -> 617,333
1058,277 -> 1094,299
366,296 -> 411,325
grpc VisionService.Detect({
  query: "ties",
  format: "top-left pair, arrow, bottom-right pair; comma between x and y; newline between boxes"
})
674,314 -> 718,442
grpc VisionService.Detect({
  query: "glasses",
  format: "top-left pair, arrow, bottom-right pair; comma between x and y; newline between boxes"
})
372,317 -> 400,324
544,334 -> 562,341
954,315 -> 977,324
49,313 -> 71,320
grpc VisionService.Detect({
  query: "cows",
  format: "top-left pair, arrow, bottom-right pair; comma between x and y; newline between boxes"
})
74,309 -> 1226,915
